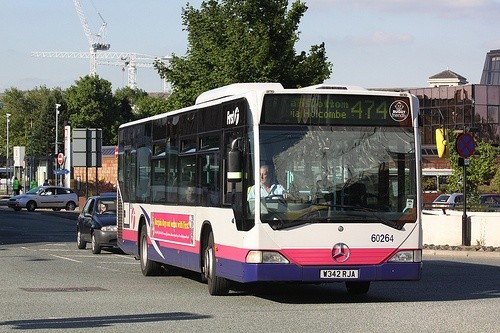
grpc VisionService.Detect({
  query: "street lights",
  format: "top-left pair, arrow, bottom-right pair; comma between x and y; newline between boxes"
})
55,104 -> 61,186
6,113 -> 11,160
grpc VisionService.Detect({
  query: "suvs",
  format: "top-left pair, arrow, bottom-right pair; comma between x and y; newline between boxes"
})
432,193 -> 462,210
7,186 -> 79,212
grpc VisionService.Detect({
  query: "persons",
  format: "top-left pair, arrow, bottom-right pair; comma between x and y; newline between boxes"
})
43,179 -> 49,186
12,177 -> 22,195
246,165 -> 291,217
98,205 -> 108,214
29,178 -> 38,189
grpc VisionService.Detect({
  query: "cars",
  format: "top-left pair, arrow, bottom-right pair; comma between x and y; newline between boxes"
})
478,193 -> 500,208
76,192 -> 118,254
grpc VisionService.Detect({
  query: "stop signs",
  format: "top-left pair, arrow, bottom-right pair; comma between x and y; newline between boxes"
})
58,153 -> 64,165
115,147 -> 119,159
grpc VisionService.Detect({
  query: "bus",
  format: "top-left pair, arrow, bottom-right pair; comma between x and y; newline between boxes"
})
349,169 -> 466,193
120,83 -> 423,297
0,168 -> 14,184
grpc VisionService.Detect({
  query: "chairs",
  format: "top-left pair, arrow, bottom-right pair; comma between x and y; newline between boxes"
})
96,203 -> 108,214
126,172 -> 222,205
